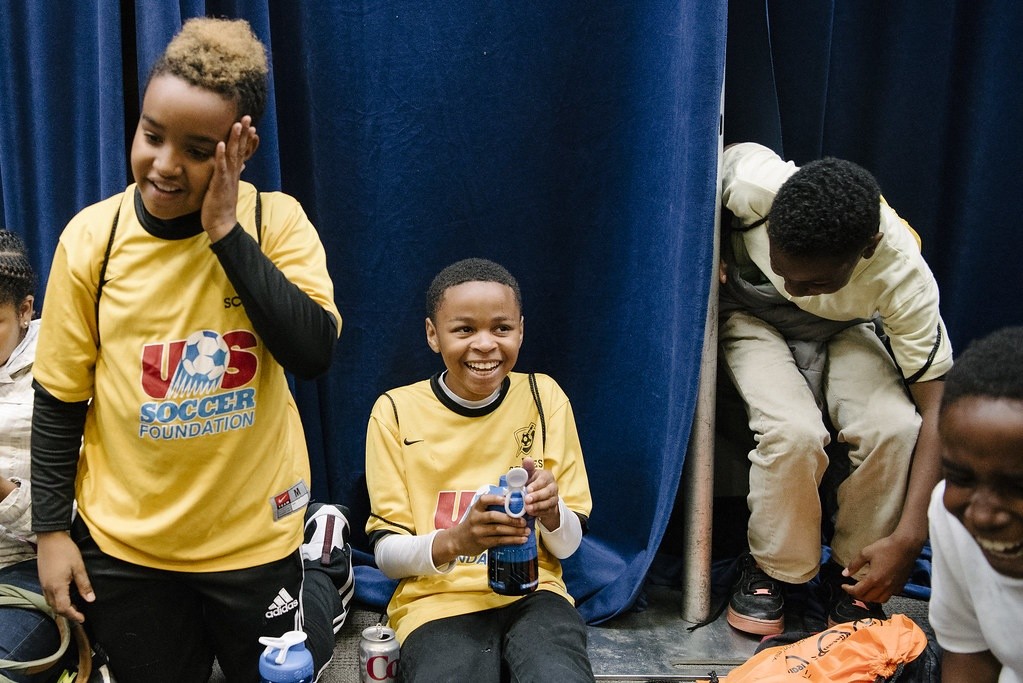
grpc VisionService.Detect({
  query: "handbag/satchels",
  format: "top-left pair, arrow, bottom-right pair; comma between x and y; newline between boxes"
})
696,613 -> 928,683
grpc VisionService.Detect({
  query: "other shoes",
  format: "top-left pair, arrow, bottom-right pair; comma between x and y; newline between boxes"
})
304,503 -> 349,563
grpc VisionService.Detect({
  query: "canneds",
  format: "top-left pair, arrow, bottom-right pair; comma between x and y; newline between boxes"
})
359,623 -> 400,683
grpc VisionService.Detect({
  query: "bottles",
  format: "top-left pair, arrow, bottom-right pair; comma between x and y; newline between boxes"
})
258,630 -> 314,683
487,468 -> 539,596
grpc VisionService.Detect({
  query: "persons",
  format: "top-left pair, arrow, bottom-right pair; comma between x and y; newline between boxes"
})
27,13 -> 356,682
721,139 -> 955,633
365,258 -> 597,683
0,226 -> 86,682
927,323 -> 1023,683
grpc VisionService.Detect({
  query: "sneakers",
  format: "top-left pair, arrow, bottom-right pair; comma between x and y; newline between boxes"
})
817,558 -> 888,630
686,553 -> 785,636
